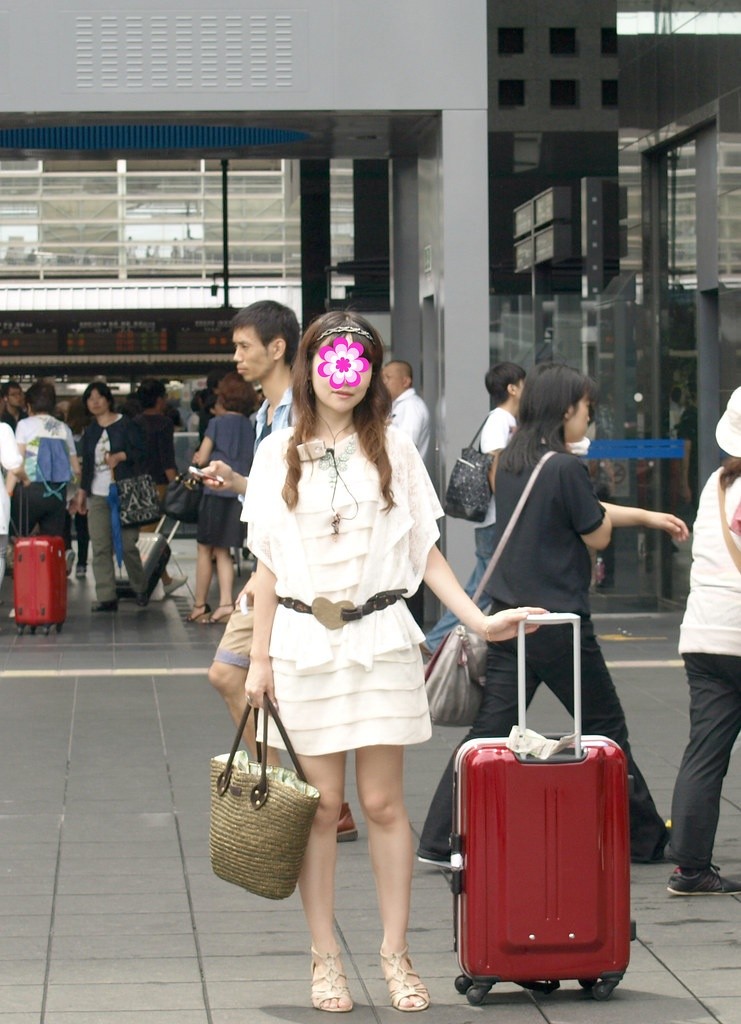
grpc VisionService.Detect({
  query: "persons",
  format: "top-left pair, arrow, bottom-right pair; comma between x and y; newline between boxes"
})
191,300 -> 360,842
419,363 -> 527,659
586,367 -> 697,601
664,384 -> 741,896
244,309 -> 550,1015
377,359 -> 430,460
417,358 -> 692,868
0,369 -> 264,626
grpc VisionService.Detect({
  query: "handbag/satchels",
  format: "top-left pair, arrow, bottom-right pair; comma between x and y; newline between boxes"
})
163,463 -> 203,525
117,452 -> 161,529
443,446 -> 495,522
424,624 -> 488,727
209,692 -> 321,900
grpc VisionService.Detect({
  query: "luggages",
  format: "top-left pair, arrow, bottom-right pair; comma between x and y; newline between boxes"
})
112,514 -> 181,599
15,480 -> 67,636
449,614 -> 636,1004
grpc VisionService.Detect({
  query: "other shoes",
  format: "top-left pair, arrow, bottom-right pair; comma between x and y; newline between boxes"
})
212,547 -> 255,563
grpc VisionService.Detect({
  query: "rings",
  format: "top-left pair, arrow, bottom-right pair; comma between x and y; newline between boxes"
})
246,696 -> 252,702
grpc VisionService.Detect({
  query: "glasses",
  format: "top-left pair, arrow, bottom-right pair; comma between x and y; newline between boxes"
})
7,392 -> 24,398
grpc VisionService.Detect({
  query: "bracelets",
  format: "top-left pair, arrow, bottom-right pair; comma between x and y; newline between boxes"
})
484,621 -> 491,642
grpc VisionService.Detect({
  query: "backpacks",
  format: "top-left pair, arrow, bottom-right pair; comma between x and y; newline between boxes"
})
29,417 -> 73,482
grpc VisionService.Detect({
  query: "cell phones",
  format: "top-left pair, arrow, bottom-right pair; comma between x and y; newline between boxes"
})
188,466 -> 224,485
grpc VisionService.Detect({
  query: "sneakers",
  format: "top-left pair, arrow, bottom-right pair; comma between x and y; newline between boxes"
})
667,867 -> 741,894
664,842 -> 673,862
337,802 -> 358,841
136,592 -> 148,606
9,608 -> 16,618
419,644 -> 432,659
164,576 -> 187,595
418,855 -> 451,869
65,550 -> 87,577
92,599 -> 118,612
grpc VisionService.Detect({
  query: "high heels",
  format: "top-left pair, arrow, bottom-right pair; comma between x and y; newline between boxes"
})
311,947 -> 353,1012
187,602 -> 235,625
380,943 -> 430,1012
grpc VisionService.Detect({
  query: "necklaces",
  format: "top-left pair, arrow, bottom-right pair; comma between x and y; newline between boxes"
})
312,433 -> 356,478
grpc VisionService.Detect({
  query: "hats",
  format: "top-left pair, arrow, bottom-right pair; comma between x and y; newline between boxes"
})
716,387 -> 741,458
